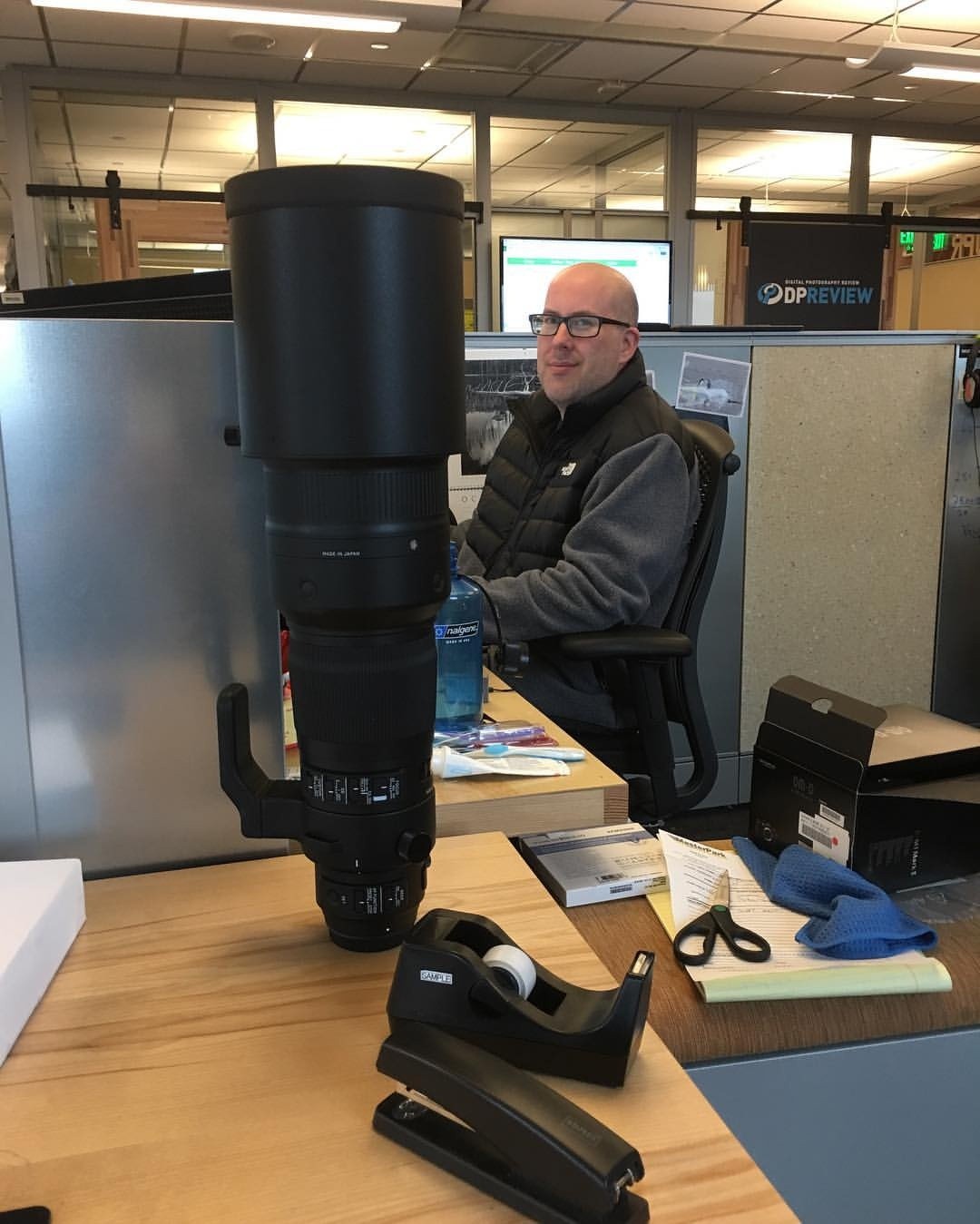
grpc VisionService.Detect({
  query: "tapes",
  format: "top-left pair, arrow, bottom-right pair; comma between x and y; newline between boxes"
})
485,933 -> 541,1000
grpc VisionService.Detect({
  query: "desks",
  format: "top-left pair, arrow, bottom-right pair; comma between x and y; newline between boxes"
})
0,830 -> 802,1224
283,663 -> 628,854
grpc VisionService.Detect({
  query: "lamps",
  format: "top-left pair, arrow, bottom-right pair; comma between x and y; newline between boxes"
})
30,0 -> 462,33
845,0 -> 980,84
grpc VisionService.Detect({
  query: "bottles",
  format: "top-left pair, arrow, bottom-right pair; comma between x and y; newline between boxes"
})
435,540 -> 484,733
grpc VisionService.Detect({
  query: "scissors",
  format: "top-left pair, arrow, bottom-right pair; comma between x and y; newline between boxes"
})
671,871 -> 773,968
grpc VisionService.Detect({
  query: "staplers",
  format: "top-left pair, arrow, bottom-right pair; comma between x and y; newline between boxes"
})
367,1023 -> 651,1221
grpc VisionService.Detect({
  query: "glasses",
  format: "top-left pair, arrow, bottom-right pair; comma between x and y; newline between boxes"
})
529,314 -> 631,338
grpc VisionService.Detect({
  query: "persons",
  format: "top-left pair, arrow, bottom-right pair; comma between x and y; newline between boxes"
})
454,263 -> 704,731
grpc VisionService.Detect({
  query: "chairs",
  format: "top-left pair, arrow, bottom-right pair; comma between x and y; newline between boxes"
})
482,419 -> 740,824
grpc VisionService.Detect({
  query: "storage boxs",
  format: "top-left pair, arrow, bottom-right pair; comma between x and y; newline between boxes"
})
747,675 -> 980,893
518,823 -> 670,907
